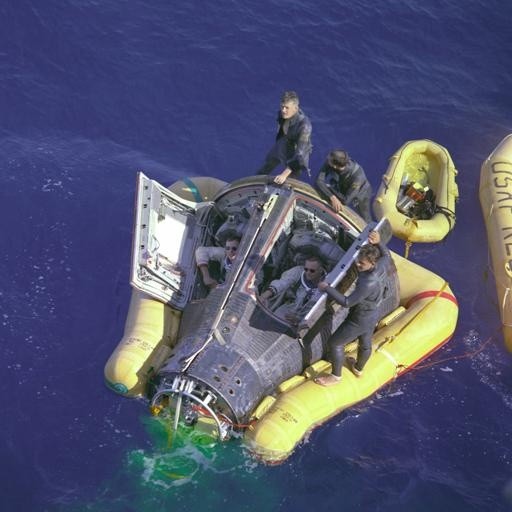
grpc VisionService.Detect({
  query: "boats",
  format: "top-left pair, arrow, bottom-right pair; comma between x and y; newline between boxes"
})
106,170 -> 458,464
478,133 -> 512,353
373,140 -> 457,243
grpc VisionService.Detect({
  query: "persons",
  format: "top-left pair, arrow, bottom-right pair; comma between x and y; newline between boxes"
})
312,230 -> 391,387
314,149 -> 373,224
195,234 -> 240,289
255,90 -> 313,185
258,256 -> 328,326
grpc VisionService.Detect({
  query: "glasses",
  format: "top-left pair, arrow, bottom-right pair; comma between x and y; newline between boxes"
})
303,267 -> 315,273
226,246 -> 238,251
